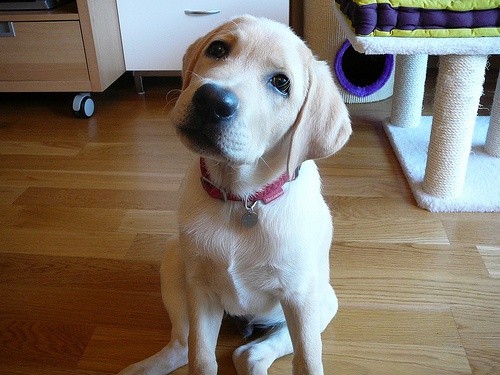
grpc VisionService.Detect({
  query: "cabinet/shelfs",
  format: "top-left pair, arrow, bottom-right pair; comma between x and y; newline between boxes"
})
116,0 -> 292,96
0,1 -> 127,121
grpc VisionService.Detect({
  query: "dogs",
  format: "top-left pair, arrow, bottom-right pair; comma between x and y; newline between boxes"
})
113,15 -> 354,375
